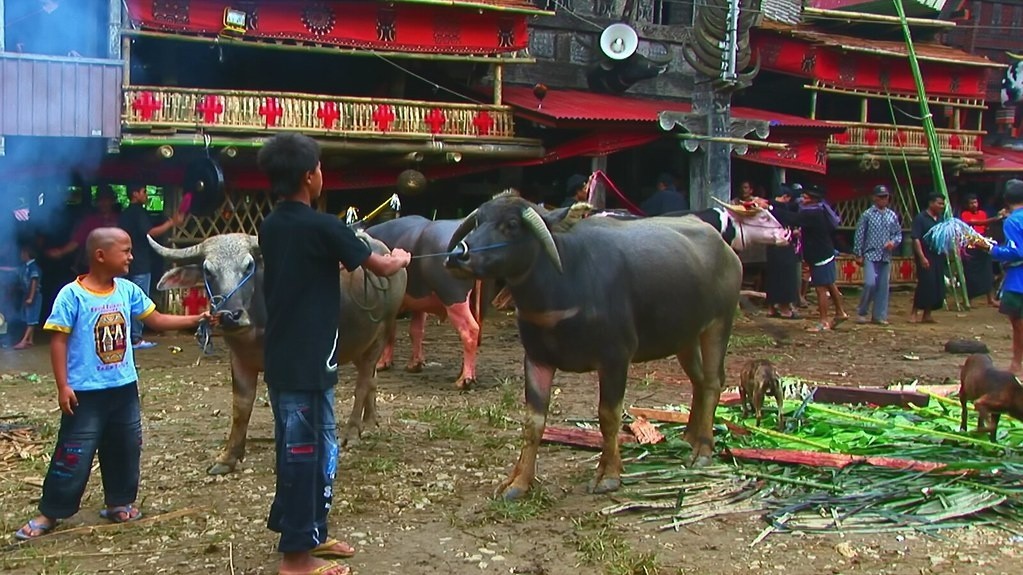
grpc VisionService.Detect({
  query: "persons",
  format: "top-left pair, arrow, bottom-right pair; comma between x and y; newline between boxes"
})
256,131 -> 412,575
906,191 -> 948,323
17,227 -> 221,539
854,184 -> 902,326
563,173 -> 852,331
962,192 -> 1000,308
1,178 -> 186,350
964,178 -> 1023,380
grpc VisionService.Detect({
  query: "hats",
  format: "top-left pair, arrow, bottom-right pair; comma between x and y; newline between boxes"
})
873,185 -> 889,196
805,184 -> 824,197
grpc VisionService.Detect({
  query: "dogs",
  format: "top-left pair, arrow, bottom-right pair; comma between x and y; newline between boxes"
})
960,352 -> 1023,441
736,356 -> 783,432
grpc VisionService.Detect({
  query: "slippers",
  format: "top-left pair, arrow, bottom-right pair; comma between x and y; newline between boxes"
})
831,317 -> 850,328
16,520 -> 50,538
781,312 -> 802,319
310,539 -> 352,556
767,312 -> 781,318
806,322 -> 830,333
100,504 -> 143,523
277,559 -> 351,575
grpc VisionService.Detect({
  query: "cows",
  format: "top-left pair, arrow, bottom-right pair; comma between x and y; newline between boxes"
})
444,193 -> 744,500
1001,50 -> 1023,106
608,201 -> 793,261
369,206 -> 483,391
143,233 -> 411,473
